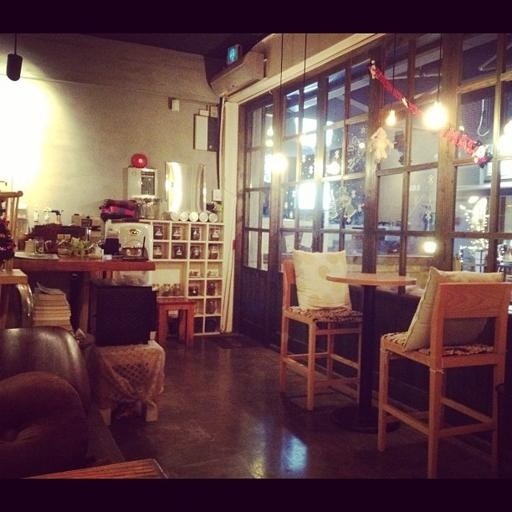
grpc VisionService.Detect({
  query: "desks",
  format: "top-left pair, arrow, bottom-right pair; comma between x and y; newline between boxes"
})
10,251 -> 156,343
322,270 -> 417,432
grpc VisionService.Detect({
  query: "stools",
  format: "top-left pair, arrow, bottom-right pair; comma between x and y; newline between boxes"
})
155,294 -> 198,349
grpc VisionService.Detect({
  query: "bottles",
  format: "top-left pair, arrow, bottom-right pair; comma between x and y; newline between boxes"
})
153,284 -> 180,297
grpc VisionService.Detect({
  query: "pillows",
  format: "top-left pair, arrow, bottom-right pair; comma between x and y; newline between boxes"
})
399,266 -> 505,353
292,245 -> 353,313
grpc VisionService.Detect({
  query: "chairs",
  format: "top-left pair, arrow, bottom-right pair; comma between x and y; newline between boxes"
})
377,281 -> 512,480
278,259 -> 361,411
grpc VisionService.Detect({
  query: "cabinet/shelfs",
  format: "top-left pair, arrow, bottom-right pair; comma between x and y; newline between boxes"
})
139,216 -> 226,339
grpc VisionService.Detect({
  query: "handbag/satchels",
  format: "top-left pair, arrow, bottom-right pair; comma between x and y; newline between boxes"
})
100,199 -> 138,220
